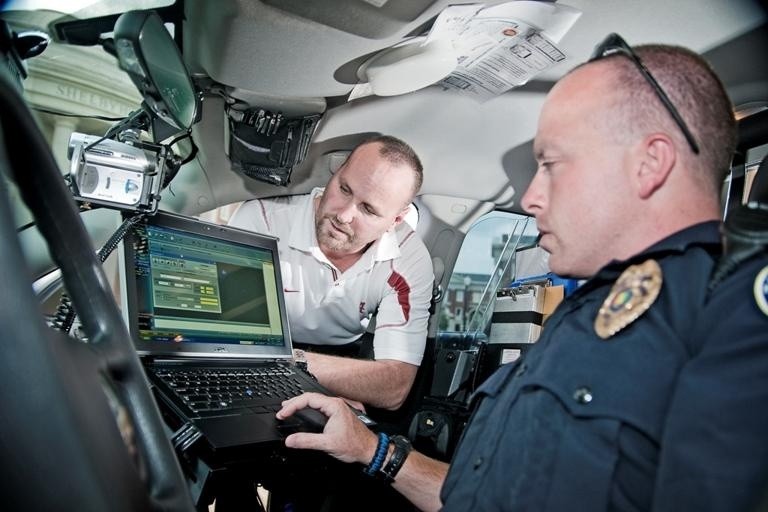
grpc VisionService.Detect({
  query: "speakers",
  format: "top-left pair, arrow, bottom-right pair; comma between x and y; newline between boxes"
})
429,348 -> 477,400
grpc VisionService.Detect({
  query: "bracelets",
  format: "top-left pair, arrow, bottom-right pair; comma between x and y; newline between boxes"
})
372,433 -> 413,489
362,431 -> 389,479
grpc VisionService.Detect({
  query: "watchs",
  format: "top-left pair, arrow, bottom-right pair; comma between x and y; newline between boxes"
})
292,346 -> 306,365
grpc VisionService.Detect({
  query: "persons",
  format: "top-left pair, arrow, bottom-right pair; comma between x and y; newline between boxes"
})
275,32 -> 768,512
205,133 -> 435,412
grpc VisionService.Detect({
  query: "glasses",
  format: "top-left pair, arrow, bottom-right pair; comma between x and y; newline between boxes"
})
590,32 -> 699,155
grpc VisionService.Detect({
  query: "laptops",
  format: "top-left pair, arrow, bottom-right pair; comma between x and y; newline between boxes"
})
116,205 -> 380,455
